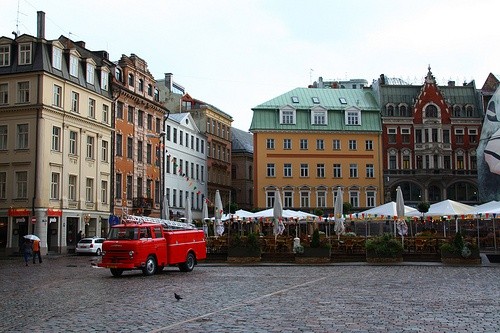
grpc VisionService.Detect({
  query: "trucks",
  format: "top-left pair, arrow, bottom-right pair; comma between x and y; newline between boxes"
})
96,220 -> 207,277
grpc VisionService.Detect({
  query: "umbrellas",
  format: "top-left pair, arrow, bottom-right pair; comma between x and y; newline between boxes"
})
395,186 -> 408,249
334,187 -> 345,246
214,189 -> 224,238
23,234 -> 41,241
273,187 -> 285,244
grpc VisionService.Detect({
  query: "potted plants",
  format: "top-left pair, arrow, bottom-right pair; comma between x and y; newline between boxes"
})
439,233 -> 482,265
367,233 -> 405,263
294,228 -> 332,264
226,229 -> 263,262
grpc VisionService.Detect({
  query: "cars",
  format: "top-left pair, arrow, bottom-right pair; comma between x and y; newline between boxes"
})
75,237 -> 106,256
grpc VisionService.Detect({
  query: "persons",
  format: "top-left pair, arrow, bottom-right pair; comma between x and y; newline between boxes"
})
76,231 -> 82,247
21,238 -> 32,266
32,240 -> 42,264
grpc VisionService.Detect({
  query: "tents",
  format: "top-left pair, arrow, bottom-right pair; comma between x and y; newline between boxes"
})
205,200 -> 500,252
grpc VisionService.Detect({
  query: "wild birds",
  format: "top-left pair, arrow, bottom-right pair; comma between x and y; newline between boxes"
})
175,294 -> 183,301
